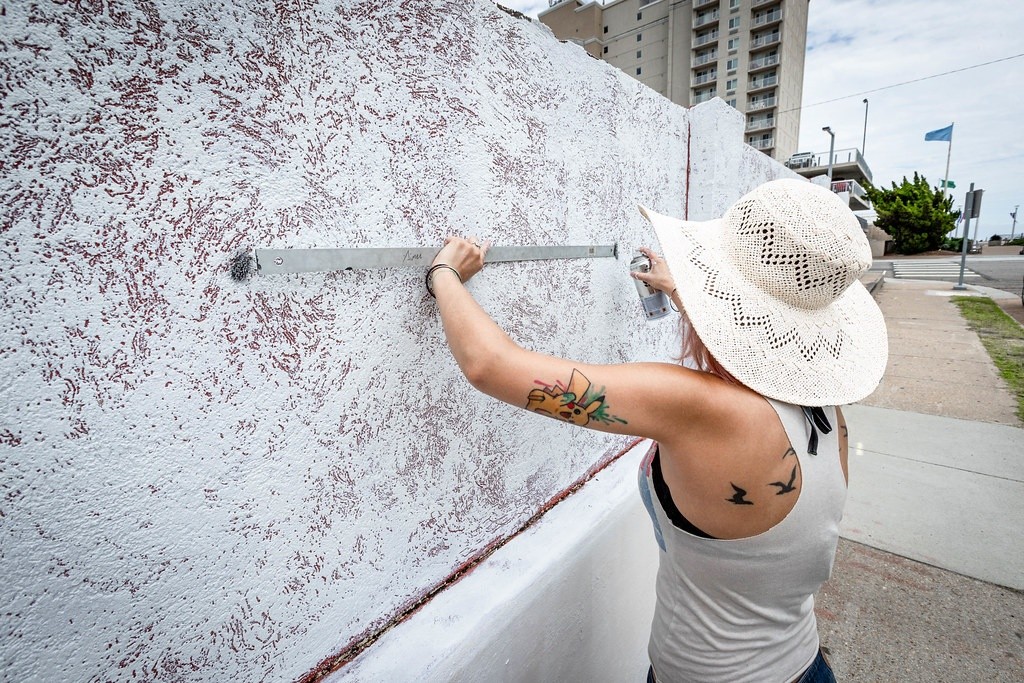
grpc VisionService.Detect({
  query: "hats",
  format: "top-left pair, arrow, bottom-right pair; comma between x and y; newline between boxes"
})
639,179 -> 889,407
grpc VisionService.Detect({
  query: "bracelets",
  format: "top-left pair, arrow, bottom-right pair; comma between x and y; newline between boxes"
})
425,264 -> 462,299
669,289 -> 680,312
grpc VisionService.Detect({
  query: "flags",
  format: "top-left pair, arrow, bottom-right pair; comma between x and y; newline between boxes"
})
925,126 -> 953,141
957,211 -> 963,223
940,179 -> 956,188
1009,213 -> 1016,222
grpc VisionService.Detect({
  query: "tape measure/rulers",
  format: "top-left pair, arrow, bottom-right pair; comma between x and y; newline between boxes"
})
256,244 -> 620,275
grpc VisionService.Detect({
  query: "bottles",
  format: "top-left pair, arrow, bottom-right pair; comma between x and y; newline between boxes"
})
631,250 -> 671,319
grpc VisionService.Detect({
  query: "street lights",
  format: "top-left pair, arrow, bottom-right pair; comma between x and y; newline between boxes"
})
862,98 -> 868,157
822,126 -> 835,182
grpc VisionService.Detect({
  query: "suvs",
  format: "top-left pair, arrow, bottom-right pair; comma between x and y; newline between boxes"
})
789,151 -> 816,167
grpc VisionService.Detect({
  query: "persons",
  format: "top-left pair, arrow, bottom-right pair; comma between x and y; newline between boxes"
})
432,178 -> 888,682
